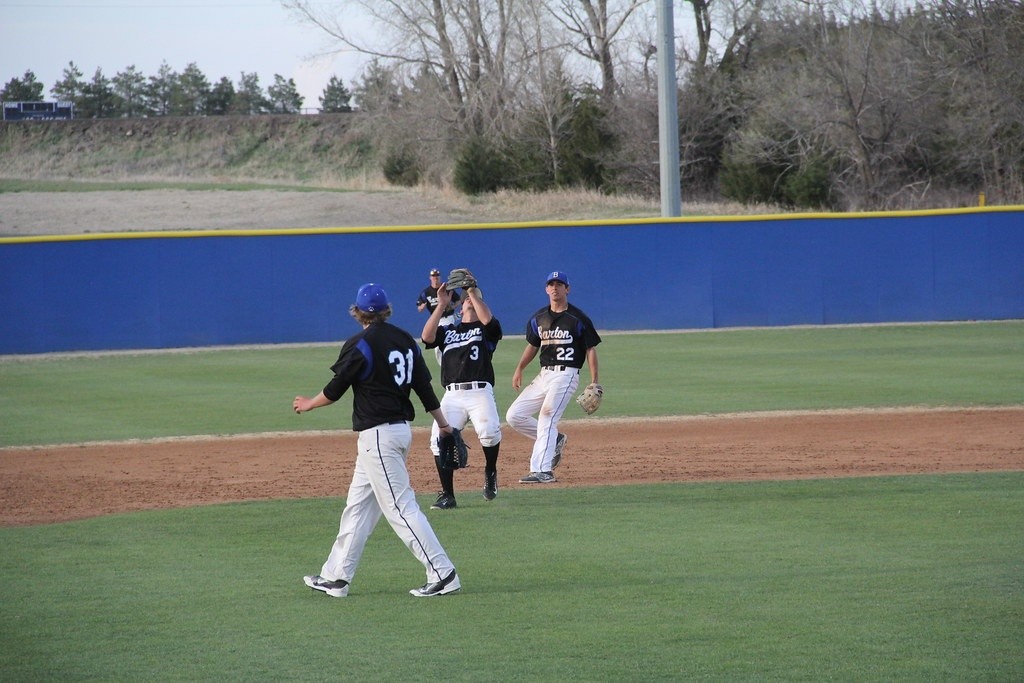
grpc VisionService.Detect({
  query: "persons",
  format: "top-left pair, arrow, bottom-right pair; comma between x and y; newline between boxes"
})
416,268 -> 461,366
421,268 -> 503,508
505,272 -> 604,484
293,283 -> 461,597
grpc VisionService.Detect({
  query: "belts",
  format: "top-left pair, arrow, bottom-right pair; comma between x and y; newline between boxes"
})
388,420 -> 406,425
544,365 -> 580,374
447,383 -> 486,391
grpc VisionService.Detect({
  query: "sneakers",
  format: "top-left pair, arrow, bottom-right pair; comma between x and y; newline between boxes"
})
409,570 -> 461,597
431,491 -> 457,509
550,432 -> 566,469
519,472 -> 560,483
303,575 -> 349,597
483,469 -> 497,500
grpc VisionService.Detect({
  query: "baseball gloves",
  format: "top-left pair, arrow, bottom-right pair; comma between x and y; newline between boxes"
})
576,383 -> 604,415
445,267 -> 478,304
436,426 -> 472,471
444,302 -> 454,313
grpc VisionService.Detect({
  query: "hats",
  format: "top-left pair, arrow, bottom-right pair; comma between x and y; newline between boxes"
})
544,272 -> 569,287
459,287 -> 483,303
431,267 -> 441,276
355,284 -> 395,313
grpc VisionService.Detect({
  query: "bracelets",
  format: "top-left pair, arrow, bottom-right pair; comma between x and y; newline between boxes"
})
439,424 -> 449,428
